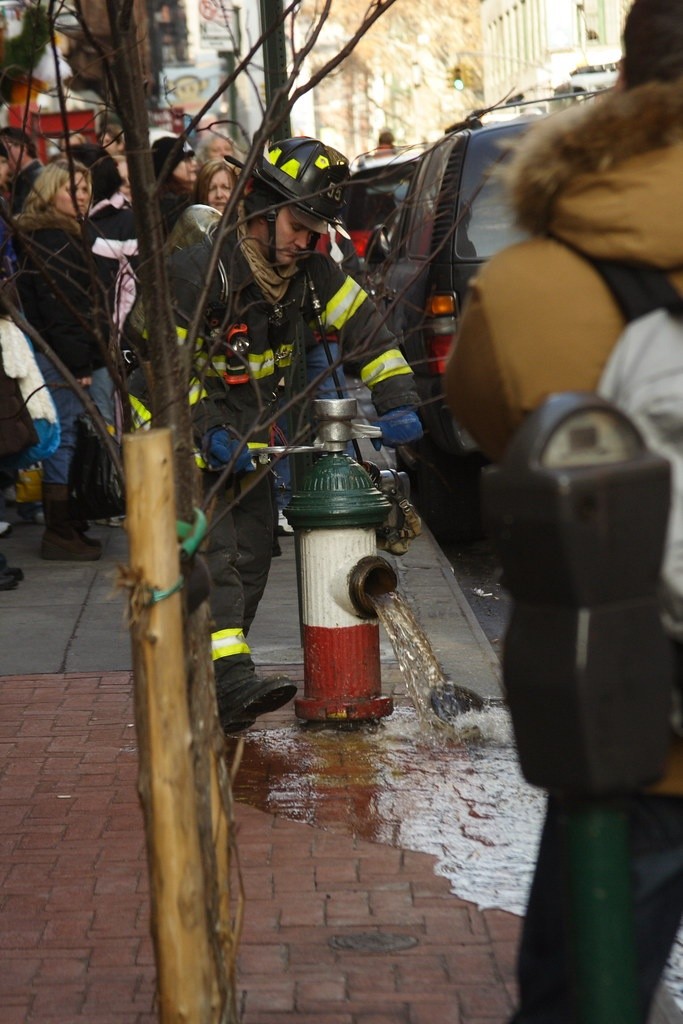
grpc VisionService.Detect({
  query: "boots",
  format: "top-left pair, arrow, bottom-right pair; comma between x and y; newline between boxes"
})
41,483 -> 102,560
70,449 -> 126,519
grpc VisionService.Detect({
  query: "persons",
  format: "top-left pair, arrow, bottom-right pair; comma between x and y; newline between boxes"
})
64,140 -> 148,394
440,0 -> 683,1023
118,134 -> 423,732
0,123 -> 142,217
0,136 -> 27,593
147,136 -> 201,239
374,132 -> 399,164
11,157 -> 119,567
192,128 -> 245,170
195,159 -> 240,214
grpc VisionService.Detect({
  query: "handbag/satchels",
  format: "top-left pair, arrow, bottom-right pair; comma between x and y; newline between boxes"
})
16,462 -> 44,503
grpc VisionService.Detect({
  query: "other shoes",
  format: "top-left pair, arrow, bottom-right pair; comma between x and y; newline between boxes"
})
218,676 -> 297,733
0,522 -> 13,537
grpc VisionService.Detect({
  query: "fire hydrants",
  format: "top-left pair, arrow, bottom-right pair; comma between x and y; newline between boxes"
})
283,397 -> 399,731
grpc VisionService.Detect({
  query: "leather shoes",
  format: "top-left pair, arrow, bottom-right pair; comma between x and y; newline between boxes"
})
3,566 -> 24,581
0,575 -> 19,590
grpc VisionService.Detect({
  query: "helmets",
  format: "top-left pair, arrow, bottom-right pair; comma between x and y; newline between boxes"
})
223,136 -> 351,224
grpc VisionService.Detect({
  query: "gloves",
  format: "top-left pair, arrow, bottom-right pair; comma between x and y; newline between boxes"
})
370,404 -> 424,451
202,427 -> 256,473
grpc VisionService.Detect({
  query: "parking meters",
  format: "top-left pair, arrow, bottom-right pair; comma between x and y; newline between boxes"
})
495,390 -> 676,1023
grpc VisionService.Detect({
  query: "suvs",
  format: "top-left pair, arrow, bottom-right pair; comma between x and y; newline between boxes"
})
364,88 -> 603,542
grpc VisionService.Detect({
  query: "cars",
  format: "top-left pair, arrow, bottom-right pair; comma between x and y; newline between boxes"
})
315,151 -> 420,271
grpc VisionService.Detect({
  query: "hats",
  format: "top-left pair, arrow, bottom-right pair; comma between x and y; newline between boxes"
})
152,138 -> 194,175
